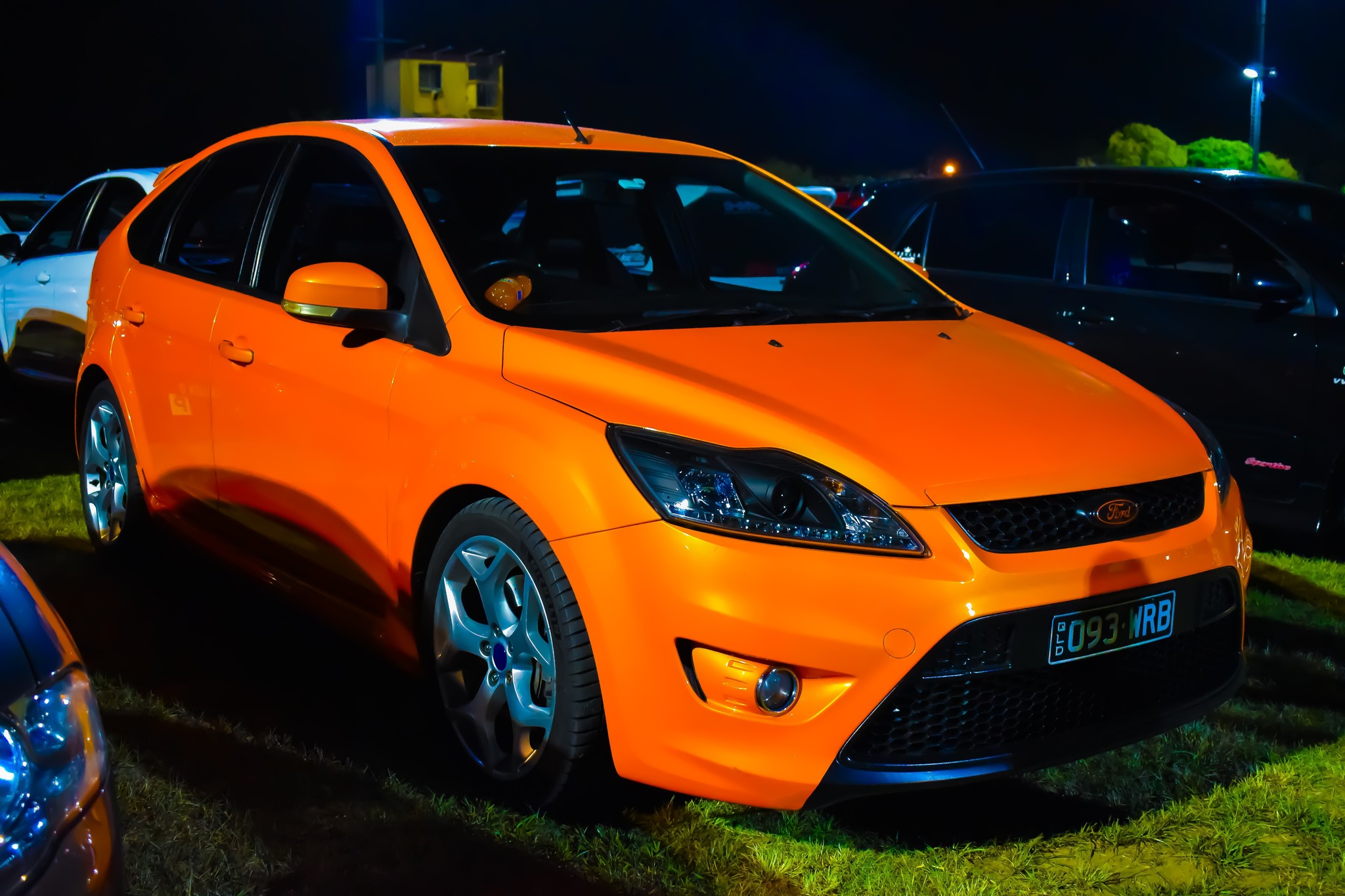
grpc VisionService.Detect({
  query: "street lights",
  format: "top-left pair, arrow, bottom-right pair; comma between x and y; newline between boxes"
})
1243,63 -> 1276,173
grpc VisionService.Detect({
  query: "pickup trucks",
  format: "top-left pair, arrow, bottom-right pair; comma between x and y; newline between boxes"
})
789,113 -> 1345,557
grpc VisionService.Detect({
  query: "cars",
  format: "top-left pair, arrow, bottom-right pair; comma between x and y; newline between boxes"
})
0,191 -> 127,266
0,543 -> 118,896
68,112 -> 1255,809
0,166 -> 168,384
